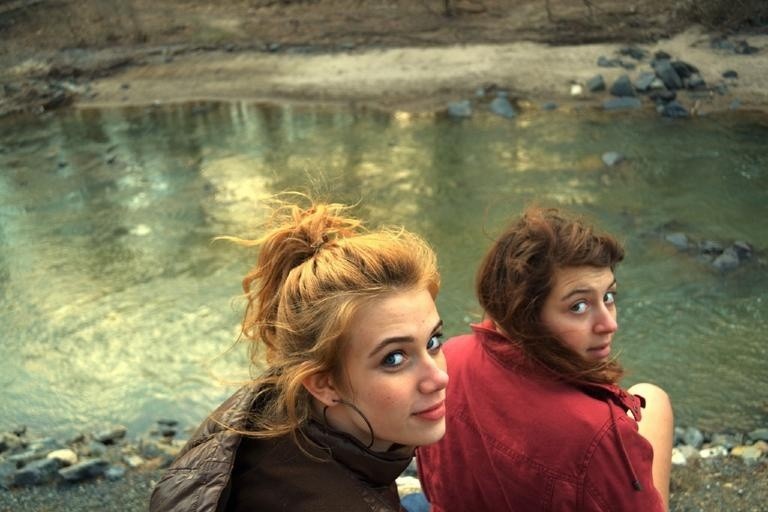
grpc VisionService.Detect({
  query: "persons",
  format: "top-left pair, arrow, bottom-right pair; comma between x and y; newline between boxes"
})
149,189 -> 450,511
414,199 -> 674,511
